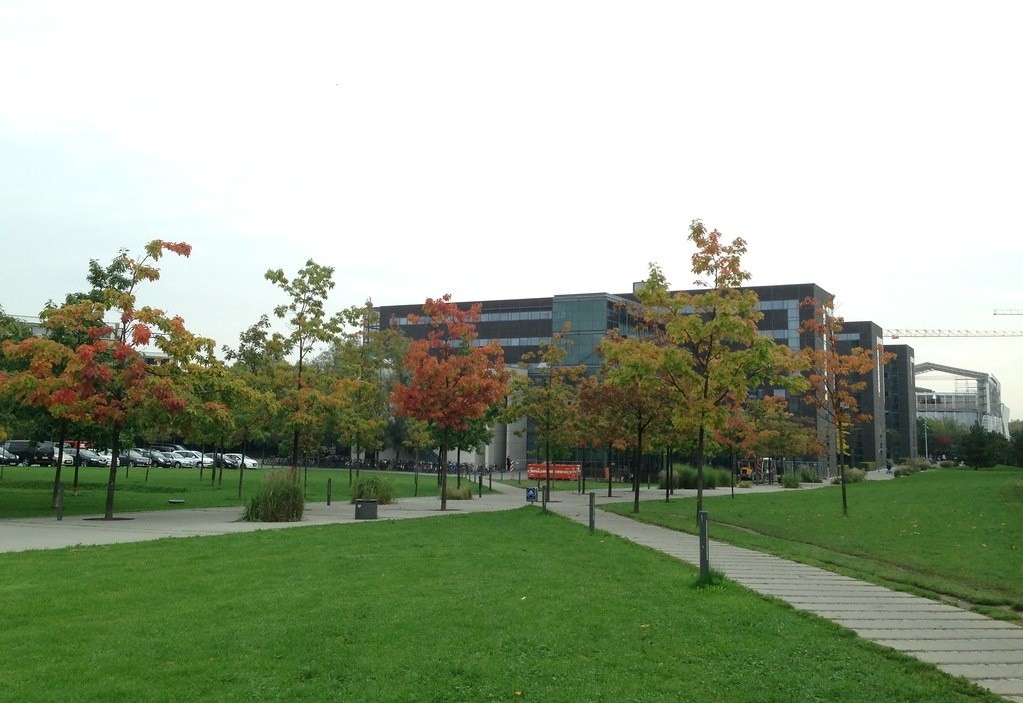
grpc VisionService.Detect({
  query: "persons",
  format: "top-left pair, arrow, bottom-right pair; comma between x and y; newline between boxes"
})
887,464 -> 891,474
507,456 -> 511,471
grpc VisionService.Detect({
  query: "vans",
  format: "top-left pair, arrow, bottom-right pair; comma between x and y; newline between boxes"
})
172,450 -> 215,469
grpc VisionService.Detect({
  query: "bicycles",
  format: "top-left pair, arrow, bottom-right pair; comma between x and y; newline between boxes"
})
262,454 -> 497,476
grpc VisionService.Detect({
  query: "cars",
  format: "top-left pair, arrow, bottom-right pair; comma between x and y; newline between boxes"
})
205,452 -> 239,469
224,453 -> 259,470
0,439 -> 188,467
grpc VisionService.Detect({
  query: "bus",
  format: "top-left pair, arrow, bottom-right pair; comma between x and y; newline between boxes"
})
526,463 -> 583,481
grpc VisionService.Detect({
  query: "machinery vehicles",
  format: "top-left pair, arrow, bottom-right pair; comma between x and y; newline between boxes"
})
738,449 -> 763,481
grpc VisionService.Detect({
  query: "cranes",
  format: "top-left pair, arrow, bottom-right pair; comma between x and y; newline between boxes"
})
882,327 -> 1022,337
